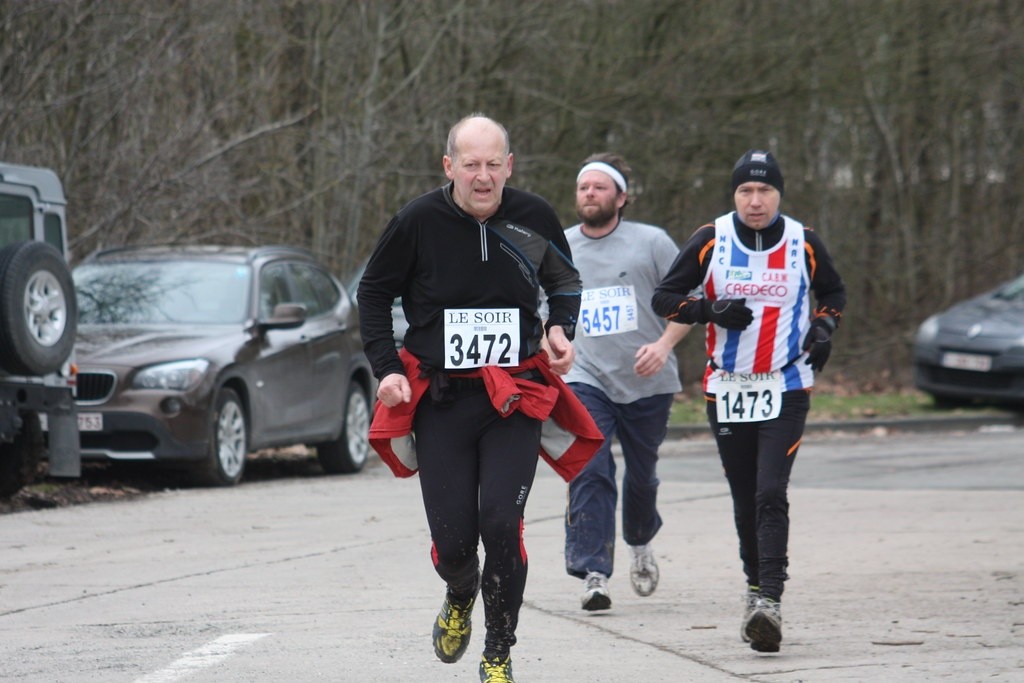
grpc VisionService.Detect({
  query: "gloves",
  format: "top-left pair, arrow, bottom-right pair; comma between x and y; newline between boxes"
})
702,296 -> 755,331
802,317 -> 833,373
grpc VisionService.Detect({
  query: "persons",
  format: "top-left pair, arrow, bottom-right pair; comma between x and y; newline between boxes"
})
653,145 -> 846,652
542,153 -> 705,611
355,112 -> 582,683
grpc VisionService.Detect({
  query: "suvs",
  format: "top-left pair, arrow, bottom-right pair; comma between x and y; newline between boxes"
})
0,159 -> 82,502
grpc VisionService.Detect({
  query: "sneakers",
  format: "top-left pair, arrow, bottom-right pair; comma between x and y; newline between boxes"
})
746,595 -> 783,652
479,648 -> 515,683
739,590 -> 759,643
432,566 -> 482,664
628,542 -> 659,595
579,568 -> 611,611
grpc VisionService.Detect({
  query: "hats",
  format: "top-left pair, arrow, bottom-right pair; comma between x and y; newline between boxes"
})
731,149 -> 784,199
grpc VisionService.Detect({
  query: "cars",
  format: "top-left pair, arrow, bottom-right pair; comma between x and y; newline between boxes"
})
69,240 -> 379,489
910,273 -> 1024,419
348,254 -> 411,366
